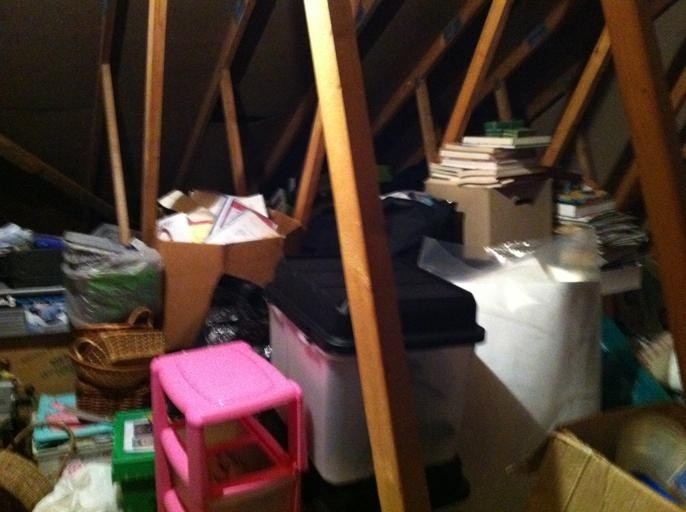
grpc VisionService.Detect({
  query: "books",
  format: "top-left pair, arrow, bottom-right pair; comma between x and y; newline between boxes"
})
32,392 -> 115,474
1,307 -> 31,337
156,189 -> 287,246
554,168 -> 658,296
425,135 -> 554,188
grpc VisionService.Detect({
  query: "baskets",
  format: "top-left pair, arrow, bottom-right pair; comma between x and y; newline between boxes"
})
0,419 -> 77,511
68,305 -> 167,425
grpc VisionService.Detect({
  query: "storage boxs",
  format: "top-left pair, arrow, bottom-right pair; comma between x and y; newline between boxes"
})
156,191 -> 302,349
112,409 -> 156,512
523,402 -> 686,512
425,180 -> 554,262
263,257 -> 485,484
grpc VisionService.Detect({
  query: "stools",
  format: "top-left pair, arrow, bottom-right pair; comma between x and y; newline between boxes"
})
150,339 -> 308,510
